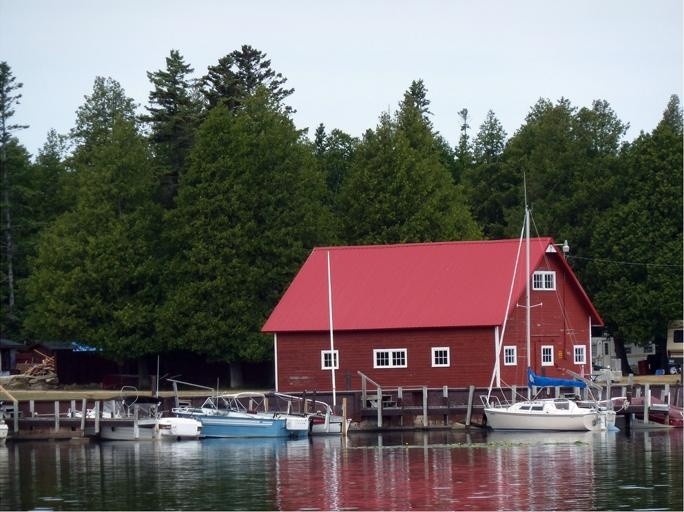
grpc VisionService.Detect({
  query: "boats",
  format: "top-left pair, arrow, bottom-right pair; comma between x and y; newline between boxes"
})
613,396 -> 683,427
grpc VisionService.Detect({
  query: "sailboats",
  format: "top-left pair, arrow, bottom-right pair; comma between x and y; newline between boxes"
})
480,172 -> 616,431
